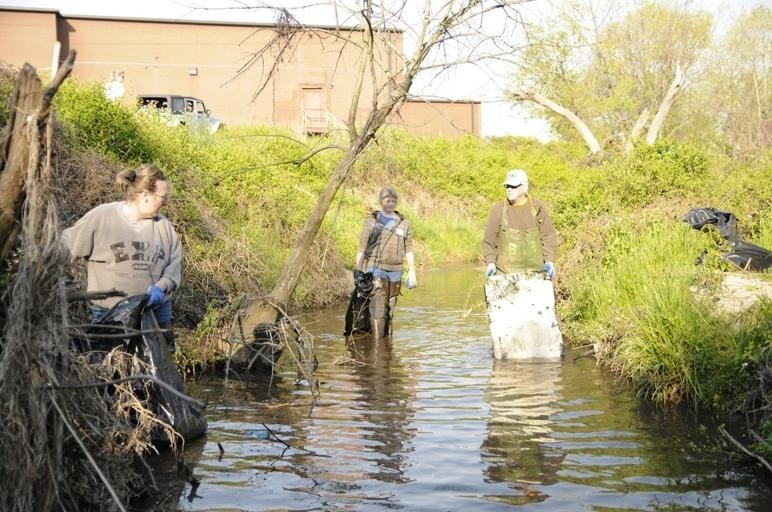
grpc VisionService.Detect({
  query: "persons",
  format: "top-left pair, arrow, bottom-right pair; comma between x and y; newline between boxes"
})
355,189 -> 417,339
482,170 -> 556,281
62,165 -> 182,364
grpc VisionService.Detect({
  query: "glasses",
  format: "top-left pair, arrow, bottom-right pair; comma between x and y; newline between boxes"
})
506,184 -> 522,190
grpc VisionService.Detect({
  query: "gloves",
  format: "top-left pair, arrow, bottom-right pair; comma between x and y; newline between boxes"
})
145,284 -> 168,309
483,263 -> 497,279
543,261 -> 556,279
403,274 -> 417,290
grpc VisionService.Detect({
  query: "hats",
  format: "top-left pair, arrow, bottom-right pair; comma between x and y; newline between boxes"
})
501,169 -> 529,186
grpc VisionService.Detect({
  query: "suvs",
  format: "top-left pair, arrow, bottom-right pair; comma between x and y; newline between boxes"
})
136,95 -> 225,133
